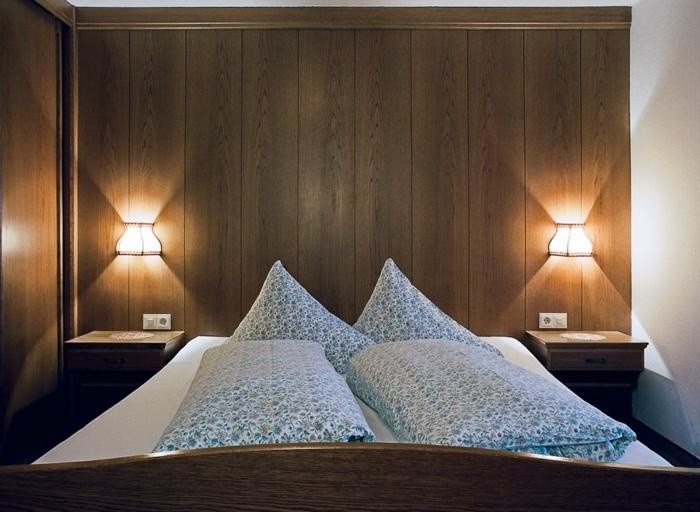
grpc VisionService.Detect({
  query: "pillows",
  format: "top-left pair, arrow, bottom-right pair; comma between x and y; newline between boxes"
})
360,259 -> 506,359
229,261 -> 373,373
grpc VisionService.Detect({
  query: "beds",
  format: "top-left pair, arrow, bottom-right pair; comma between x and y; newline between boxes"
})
0,335 -> 700,512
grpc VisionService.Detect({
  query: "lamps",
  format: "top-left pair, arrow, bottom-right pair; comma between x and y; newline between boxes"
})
548,220 -> 595,258
114,220 -> 163,257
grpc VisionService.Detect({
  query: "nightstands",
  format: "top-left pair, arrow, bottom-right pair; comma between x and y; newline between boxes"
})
60,330 -> 188,428
527,329 -> 650,426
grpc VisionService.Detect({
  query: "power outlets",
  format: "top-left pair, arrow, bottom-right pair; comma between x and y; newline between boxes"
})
141,314 -> 172,331
538,312 -> 567,329
